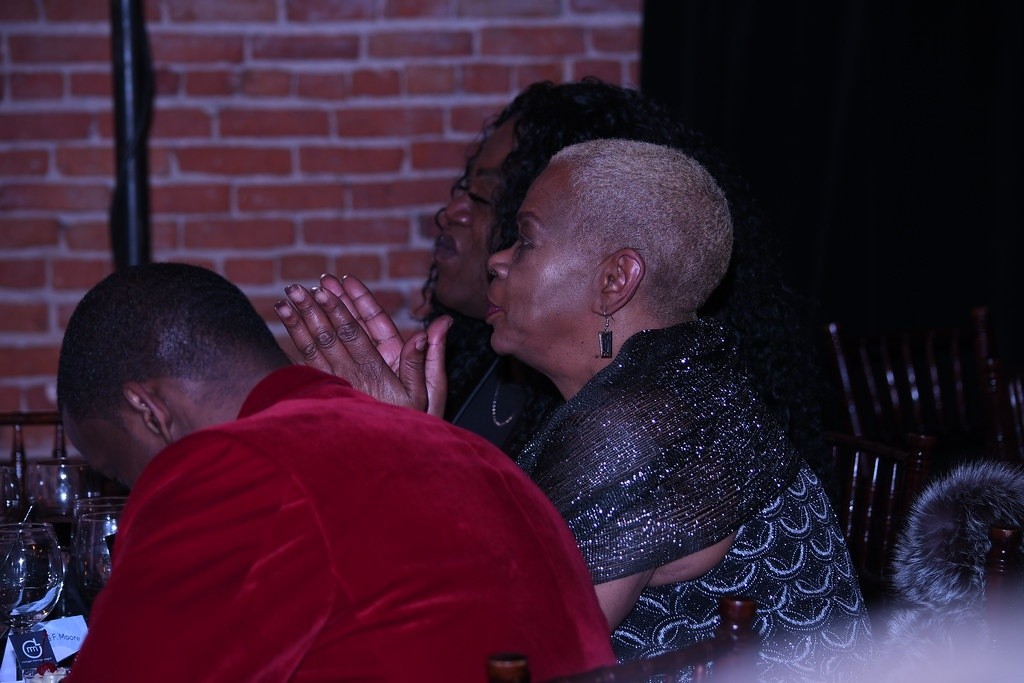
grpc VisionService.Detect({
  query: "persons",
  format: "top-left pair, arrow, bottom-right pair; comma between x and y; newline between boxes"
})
275,134 -> 876,682
408,76 -> 689,422
53,261 -> 617,682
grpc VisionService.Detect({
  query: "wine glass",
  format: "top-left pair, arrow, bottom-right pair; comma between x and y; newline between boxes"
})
0,521 -> 64,683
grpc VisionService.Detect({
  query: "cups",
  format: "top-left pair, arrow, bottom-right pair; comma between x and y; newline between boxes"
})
0,457 -> 133,629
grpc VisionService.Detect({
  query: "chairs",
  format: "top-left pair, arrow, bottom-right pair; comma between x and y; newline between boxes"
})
823,429 -> 935,602
821,306 -> 999,462
486,594 -> 763,683
885,452 -> 1024,682
